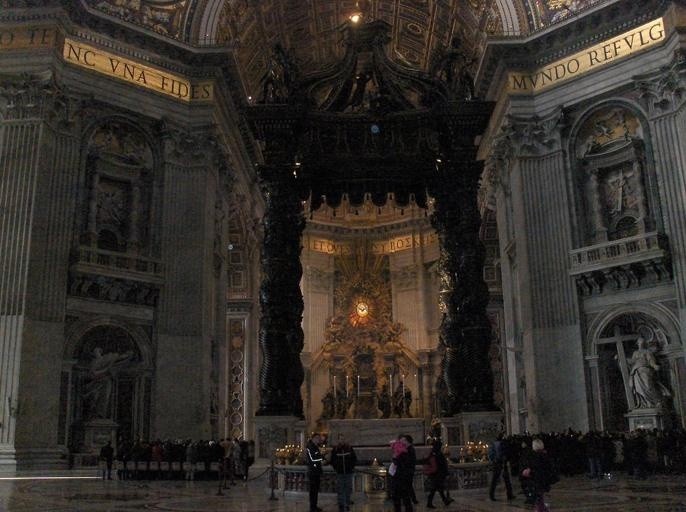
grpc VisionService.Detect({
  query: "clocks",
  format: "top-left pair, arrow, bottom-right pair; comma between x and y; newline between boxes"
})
356,302 -> 369,317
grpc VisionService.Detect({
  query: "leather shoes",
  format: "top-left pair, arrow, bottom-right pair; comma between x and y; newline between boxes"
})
489,494 -> 517,501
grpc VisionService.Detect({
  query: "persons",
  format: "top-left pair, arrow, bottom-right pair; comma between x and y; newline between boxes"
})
86,345 -> 125,420
328,432 -> 358,512
515,437 -> 535,505
487,432 -> 517,502
506,425 -> 686,479
97,434 -> 256,483
304,432 -> 331,512
613,336 -> 668,410
388,435 -> 419,506
387,435 -> 417,512
423,440 -> 455,510
388,434 -> 410,475
522,437 -> 553,511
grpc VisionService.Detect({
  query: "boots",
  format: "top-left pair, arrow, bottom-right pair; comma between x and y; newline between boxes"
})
427,497 -> 454,509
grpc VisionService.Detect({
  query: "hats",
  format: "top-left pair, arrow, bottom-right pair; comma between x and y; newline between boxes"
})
399,434 -> 413,444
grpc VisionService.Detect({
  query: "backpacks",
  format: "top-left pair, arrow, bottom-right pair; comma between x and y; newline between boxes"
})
424,457 -> 438,475
545,460 -> 560,485
